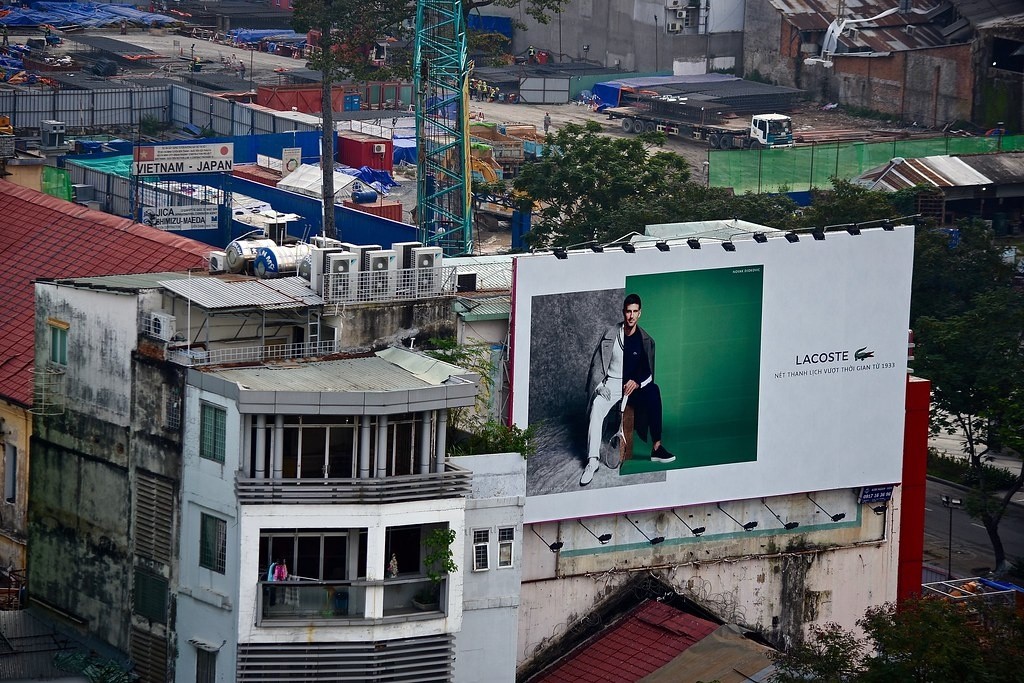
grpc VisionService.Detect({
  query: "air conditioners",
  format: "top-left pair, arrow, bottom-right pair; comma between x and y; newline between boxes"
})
667,22 -> 680,32
263,222 -> 288,242
451,271 -> 478,292
373,144 -> 386,153
150,310 -> 176,342
324,252 -> 359,301
849,27 -> 859,42
408,246 -> 443,296
677,11 -> 687,18
352,244 -> 382,300
310,237 -> 358,253
209,250 -> 233,273
309,247 -> 343,296
906,25 -> 916,37
365,249 -> 398,299
392,240 -> 423,293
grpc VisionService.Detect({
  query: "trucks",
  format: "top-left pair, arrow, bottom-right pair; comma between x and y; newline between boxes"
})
505,125 -> 560,162
438,144 -> 503,182
468,121 -> 524,179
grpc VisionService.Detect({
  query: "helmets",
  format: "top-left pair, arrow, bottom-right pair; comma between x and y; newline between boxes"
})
478,80 -> 482,84
471,78 -> 475,82
483,82 -> 486,85
546,111 -> 549,114
470,83 -> 472,85
240,60 -> 243,63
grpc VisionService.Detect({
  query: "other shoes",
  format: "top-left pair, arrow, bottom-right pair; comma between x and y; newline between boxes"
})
545,133 -> 549,135
470,98 -> 473,100
580,460 -> 599,485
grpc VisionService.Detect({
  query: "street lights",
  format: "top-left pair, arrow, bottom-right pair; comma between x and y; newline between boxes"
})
583,45 -> 589,76
191,44 -> 195,59
939,493 -> 962,581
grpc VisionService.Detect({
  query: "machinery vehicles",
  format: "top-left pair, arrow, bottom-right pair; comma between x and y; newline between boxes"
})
438,142 -> 540,232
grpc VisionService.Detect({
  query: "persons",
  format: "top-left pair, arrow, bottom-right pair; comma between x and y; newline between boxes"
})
232,53 -> 237,65
187,59 -> 195,71
475,80 -> 484,102
481,82 -> 489,101
0,25 -> 9,45
528,46 -> 535,64
579,293 -> 676,485
543,112 -> 551,135
44,24 -> 51,38
469,79 -> 475,100
238,60 -> 246,79
490,91 -> 496,102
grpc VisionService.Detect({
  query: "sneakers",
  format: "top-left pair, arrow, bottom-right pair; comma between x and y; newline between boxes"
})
651,446 -> 676,463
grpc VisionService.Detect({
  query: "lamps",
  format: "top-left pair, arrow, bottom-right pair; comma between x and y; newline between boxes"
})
532,213 -> 926,260
578,519 -> 612,544
761,496 -> 799,530
807,492 -> 846,522
623,514 -> 665,545
716,501 -> 758,531
670,508 -> 705,537
531,524 -> 563,554
851,487 -> 888,515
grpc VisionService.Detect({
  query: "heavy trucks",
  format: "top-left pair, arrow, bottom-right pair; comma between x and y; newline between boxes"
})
602,92 -> 793,154
